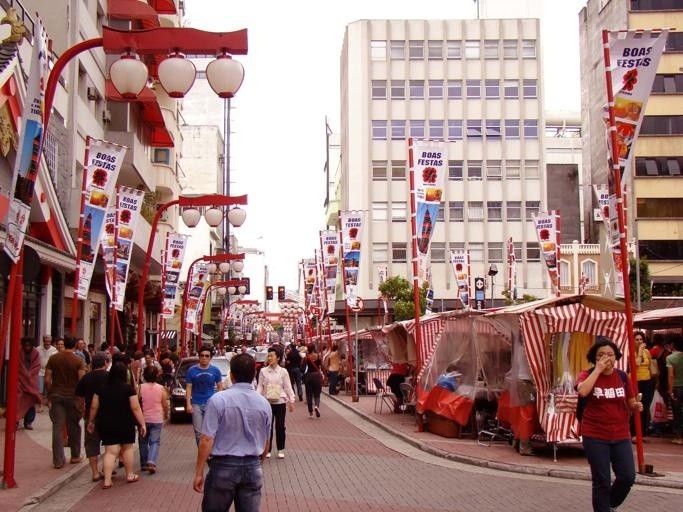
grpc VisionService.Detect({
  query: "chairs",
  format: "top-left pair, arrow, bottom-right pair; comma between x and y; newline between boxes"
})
372,378 -> 396,414
401,383 -> 417,416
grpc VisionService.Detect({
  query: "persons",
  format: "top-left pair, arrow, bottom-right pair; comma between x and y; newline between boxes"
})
17,333 -> 94,430
573,336 -> 642,511
272,339 -> 342,417
626,333 -> 682,445
385,361 -> 411,414
44,335 -> 84,468
185,346 -> 295,511
76,341 -> 178,489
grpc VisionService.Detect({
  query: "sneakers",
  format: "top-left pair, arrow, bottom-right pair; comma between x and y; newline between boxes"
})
277,450 -> 284,459
314,406 -> 319,417
265,452 -> 271,458
146,463 -> 156,474
141,464 -> 148,472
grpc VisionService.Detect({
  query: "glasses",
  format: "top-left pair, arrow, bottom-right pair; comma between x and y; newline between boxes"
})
595,353 -> 615,357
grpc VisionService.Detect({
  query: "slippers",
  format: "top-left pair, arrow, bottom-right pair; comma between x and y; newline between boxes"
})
54,457 -> 66,469
200,354 -> 209,358
92,459 -> 140,489
70,455 -> 84,464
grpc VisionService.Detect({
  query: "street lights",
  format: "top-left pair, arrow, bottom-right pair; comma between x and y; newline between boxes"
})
242,298 -> 309,345
488,264 -> 499,309
181,253 -> 244,358
2,24 -> 248,492
220,298 -> 260,351
198,280 -> 249,350
136,194 -> 248,358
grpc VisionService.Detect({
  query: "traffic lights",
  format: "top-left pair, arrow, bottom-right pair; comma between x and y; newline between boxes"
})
266,286 -> 273,300
278,286 -> 286,299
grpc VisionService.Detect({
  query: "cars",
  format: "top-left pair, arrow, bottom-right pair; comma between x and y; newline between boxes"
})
169,356 -> 234,422
254,353 -> 268,371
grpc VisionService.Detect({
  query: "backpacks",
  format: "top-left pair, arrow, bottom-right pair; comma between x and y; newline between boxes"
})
575,366 -> 627,422
262,367 -> 285,402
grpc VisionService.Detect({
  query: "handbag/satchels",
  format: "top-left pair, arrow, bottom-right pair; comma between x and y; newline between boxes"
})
643,349 -> 658,377
319,370 -> 325,384
323,370 -> 328,387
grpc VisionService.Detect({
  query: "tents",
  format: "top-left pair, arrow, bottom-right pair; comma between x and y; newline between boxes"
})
306,294 -> 636,462
